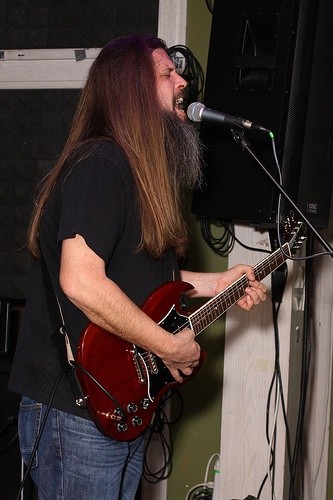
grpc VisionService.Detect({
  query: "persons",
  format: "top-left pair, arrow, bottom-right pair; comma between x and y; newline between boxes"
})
8,36 -> 270,499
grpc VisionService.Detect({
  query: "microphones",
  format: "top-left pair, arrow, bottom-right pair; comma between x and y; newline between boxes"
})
187,102 -> 268,132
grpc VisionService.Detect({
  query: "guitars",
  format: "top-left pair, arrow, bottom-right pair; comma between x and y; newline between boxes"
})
72,209 -> 310,445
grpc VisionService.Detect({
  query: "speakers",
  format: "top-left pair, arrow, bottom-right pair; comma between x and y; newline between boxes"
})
191,0 -> 333,230
